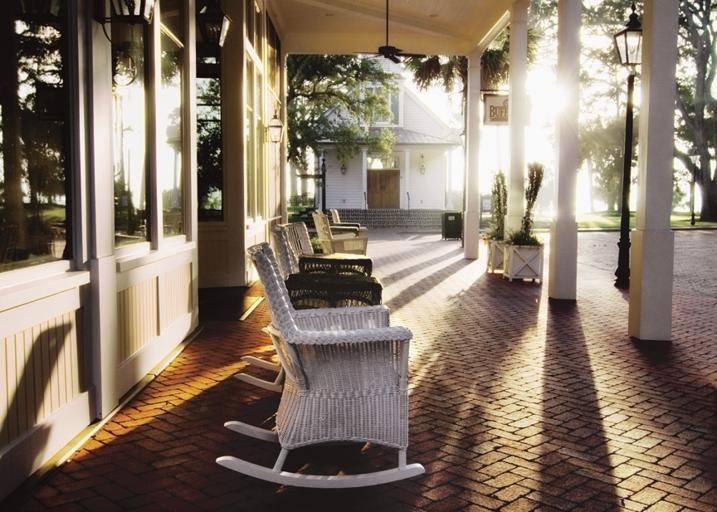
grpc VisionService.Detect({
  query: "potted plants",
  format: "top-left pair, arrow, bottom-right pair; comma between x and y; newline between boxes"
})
482,169 -> 508,273
504,161 -> 544,282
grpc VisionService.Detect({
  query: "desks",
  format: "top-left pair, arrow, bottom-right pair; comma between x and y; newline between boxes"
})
290,273 -> 384,292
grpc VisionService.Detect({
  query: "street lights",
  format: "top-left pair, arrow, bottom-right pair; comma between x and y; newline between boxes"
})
607,2 -> 644,292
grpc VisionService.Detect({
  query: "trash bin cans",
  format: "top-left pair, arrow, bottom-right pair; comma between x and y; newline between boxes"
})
442,213 -> 461,241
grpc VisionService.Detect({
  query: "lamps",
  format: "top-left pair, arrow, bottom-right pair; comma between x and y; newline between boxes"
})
266,110 -> 284,143
95,0 -> 153,52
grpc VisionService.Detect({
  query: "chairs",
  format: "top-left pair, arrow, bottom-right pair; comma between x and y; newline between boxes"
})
233,220 -> 299,396
298,255 -> 373,277
216,241 -> 426,489
283,278 -> 383,312
294,209 -> 369,255
